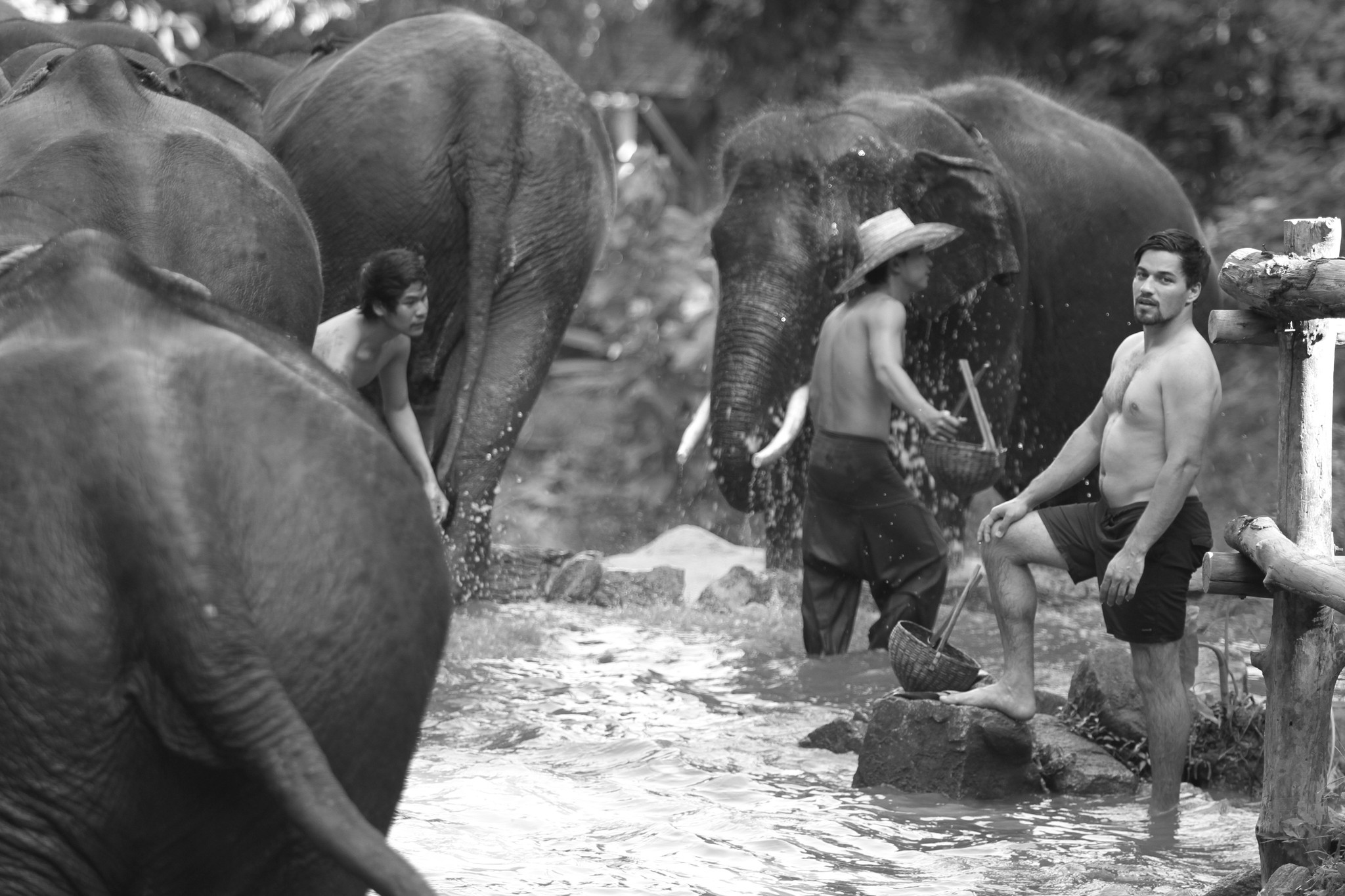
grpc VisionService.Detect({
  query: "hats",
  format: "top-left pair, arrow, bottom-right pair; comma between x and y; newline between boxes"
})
831,208 -> 965,295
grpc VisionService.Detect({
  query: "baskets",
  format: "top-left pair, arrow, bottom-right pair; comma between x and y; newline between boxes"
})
888,565 -> 984,692
921,358 -> 1007,497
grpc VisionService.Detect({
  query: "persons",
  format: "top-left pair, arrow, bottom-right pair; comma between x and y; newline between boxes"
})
312,250 -> 450,526
801,208 -> 967,658
938,229 -> 1222,815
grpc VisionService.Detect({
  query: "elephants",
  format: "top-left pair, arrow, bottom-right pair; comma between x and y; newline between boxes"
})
0,2 -> 606,896
678,76 -> 1223,577
